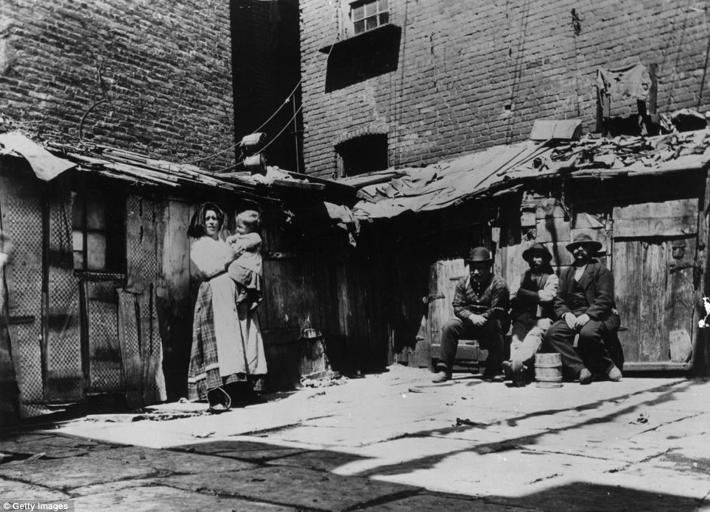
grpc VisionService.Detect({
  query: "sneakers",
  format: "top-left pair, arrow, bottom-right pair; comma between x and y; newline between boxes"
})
431,360 -> 529,387
577,366 -> 622,384
236,289 -> 264,311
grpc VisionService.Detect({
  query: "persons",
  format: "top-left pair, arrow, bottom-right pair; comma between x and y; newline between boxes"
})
225,209 -> 262,311
189,203 -> 266,407
548,233 -> 623,383
433,246 -> 510,383
502,243 -> 559,383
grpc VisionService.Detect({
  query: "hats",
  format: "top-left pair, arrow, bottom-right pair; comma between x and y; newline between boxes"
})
464,246 -> 494,265
522,242 -> 553,262
565,233 -> 602,252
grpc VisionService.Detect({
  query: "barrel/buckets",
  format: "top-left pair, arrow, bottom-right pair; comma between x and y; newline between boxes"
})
534,353 -> 563,388
